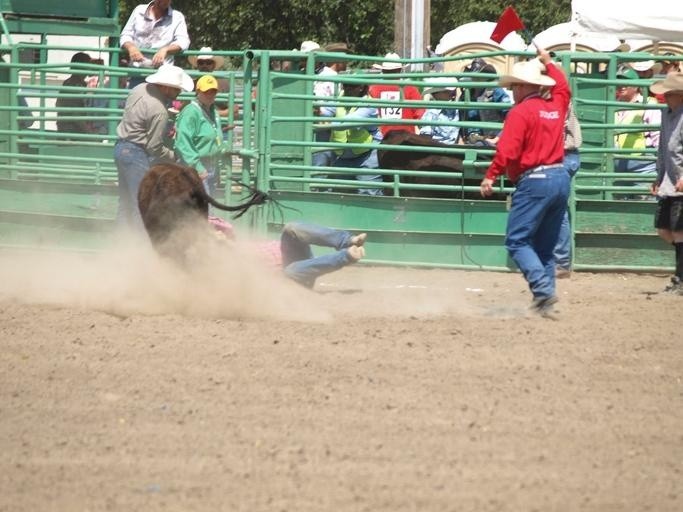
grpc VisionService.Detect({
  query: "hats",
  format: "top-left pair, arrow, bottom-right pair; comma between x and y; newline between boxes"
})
196,75 -> 219,92
294,40 -> 497,91
188,47 -> 224,69
616,69 -> 640,80
145,63 -> 194,91
649,71 -> 683,94
497,62 -> 555,89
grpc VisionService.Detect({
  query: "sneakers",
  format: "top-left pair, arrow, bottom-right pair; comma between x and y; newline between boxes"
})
554,266 -> 572,279
527,295 -> 558,315
664,277 -> 683,294
347,246 -> 367,260
353,233 -> 367,246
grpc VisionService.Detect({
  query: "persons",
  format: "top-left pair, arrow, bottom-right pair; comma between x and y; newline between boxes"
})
533,59 -> 582,281
186,211 -> 369,297
0,56 -> 36,163
53,43 -> 683,205
643,67 -> 683,291
169,74 -> 220,211
111,63 -> 194,237
477,45 -> 578,312
117,0 -> 190,88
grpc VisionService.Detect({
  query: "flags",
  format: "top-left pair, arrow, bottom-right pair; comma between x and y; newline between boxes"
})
489,6 -> 526,42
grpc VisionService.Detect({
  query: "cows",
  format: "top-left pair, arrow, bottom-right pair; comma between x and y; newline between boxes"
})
136,160 -> 303,286
376,128 -> 509,201
